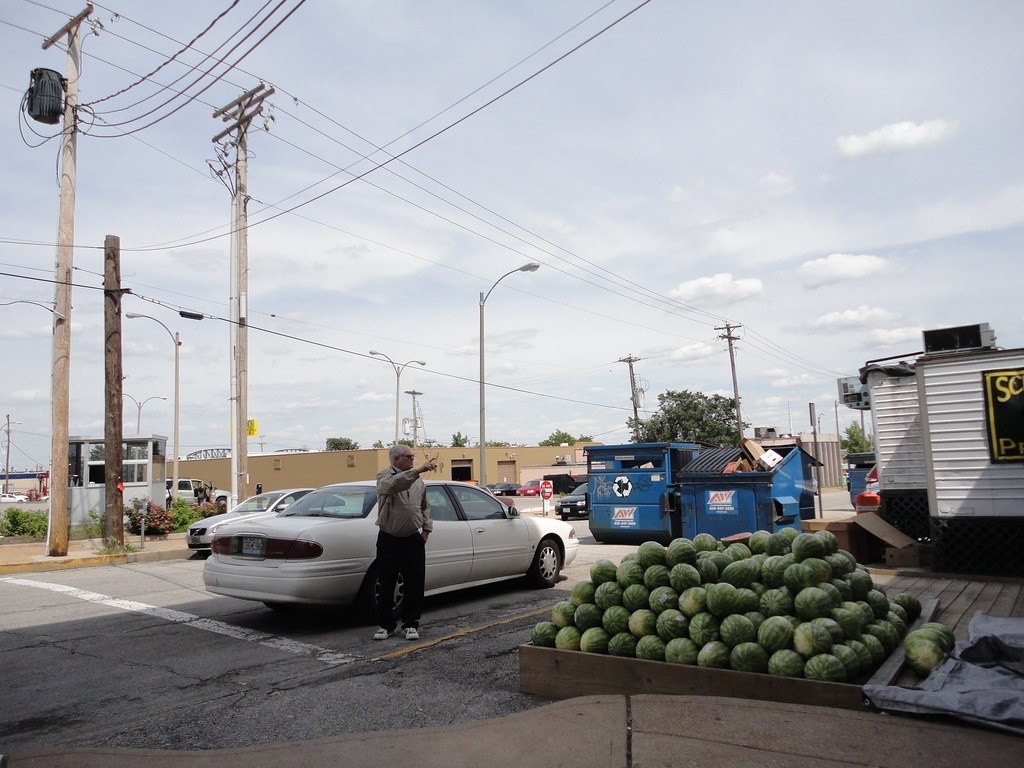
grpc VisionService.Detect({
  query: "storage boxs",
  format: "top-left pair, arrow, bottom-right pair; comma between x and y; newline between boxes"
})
800,516 -> 928,569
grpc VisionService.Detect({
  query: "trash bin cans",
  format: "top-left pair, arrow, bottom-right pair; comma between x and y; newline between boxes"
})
675,443 -> 825,541
844,451 -> 875,510
582,441 -> 701,545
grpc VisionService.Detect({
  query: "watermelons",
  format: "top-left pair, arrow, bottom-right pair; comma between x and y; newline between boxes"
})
531,527 -> 923,684
904,622 -> 955,675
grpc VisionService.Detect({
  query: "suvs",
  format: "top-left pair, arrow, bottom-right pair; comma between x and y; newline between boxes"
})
165,479 -> 230,510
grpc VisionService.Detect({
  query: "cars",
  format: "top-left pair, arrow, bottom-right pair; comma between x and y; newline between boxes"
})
555,482 -> 589,521
0,492 -> 30,504
185,489 -> 319,557
494,483 -> 521,496
516,480 -> 541,496
200,479 -> 579,623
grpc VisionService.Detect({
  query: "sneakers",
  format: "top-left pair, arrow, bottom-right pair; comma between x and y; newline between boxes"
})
402,627 -> 419,639
374,627 -> 396,639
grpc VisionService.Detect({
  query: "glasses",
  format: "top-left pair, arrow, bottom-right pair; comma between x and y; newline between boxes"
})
399,454 -> 414,459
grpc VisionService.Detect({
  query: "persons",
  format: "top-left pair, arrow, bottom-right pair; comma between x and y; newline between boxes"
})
372,445 -> 437,640
198,481 -> 209,506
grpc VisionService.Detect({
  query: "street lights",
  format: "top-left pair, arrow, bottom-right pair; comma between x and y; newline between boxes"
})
370,350 -> 426,445
480,262 -> 540,488
122,393 -> 166,436
125,312 -> 182,503
819,412 -> 826,433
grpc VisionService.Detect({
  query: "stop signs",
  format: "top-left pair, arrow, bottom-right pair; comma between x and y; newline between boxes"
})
539,481 -> 554,501
